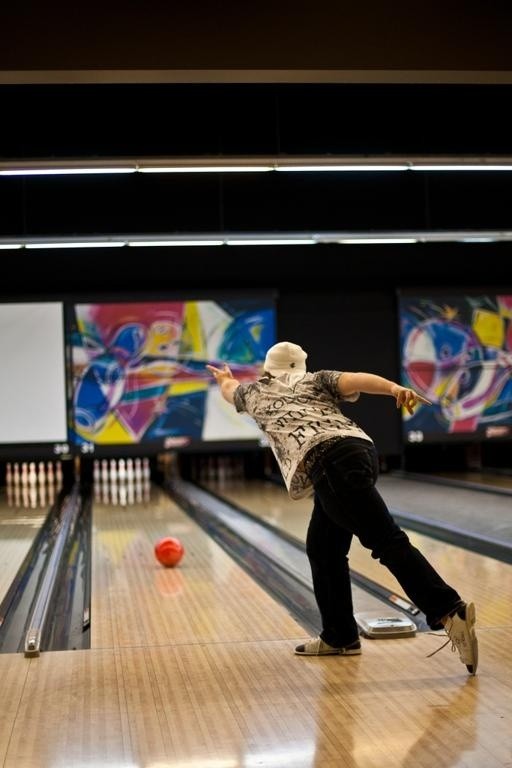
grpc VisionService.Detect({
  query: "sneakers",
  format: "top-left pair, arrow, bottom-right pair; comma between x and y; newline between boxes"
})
444,602 -> 478,676
293,637 -> 362,656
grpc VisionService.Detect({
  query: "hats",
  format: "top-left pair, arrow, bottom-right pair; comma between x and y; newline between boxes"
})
263,341 -> 308,378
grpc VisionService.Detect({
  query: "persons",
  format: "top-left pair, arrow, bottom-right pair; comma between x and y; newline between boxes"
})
205,341 -> 478,676
